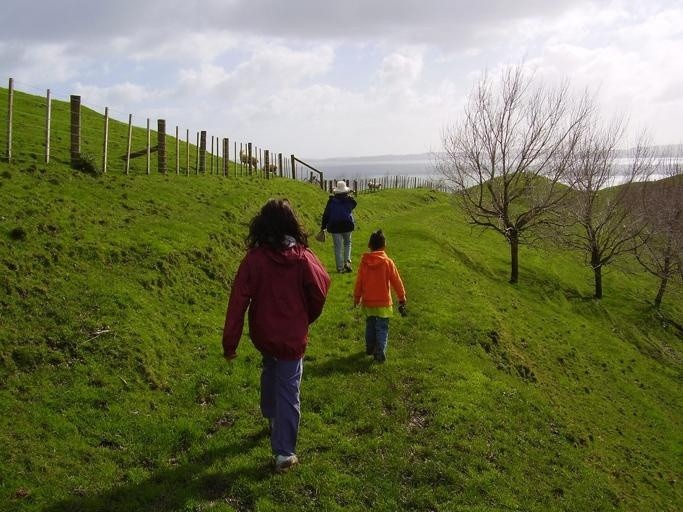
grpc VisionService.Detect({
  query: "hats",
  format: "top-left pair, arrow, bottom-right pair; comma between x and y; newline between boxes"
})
333,180 -> 350,192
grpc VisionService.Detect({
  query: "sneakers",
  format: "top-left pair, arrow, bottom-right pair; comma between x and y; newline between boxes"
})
366,347 -> 386,362
337,261 -> 353,273
275,452 -> 298,472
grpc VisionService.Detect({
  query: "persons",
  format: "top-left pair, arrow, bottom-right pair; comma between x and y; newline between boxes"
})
319,179 -> 357,273
222,198 -> 331,473
352,230 -> 408,362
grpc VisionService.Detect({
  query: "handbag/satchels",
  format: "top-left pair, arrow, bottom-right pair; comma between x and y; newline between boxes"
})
315,230 -> 326,242
